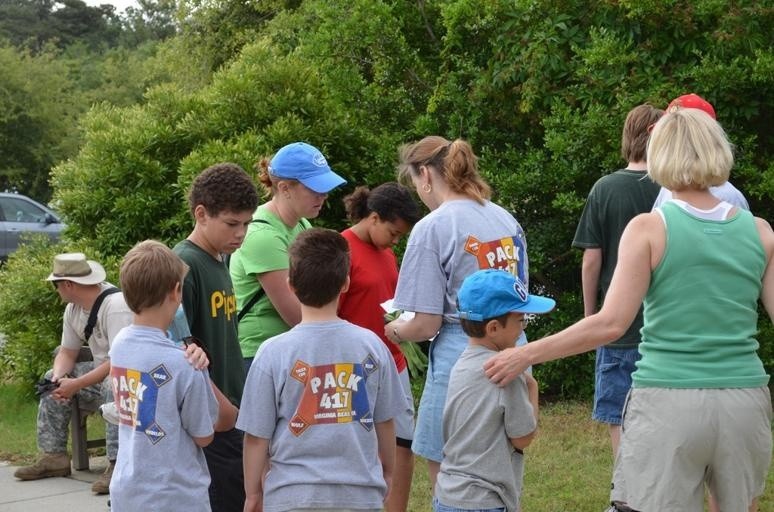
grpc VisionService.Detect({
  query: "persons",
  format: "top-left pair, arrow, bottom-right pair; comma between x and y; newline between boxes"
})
650,93 -> 752,215
107,238 -> 219,512
170,162 -> 261,512
225,141 -> 350,376
231,226 -> 410,512
570,101 -> 670,463
430,266 -> 559,511
480,104 -> 774,509
382,133 -> 529,502
337,179 -> 419,428
10,252 -> 137,494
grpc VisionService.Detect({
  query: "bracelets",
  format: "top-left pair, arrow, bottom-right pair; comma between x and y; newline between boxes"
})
392,326 -> 406,344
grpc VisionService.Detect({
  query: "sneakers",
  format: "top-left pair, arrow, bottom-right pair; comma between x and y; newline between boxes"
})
92,464 -> 114,493
15,450 -> 70,479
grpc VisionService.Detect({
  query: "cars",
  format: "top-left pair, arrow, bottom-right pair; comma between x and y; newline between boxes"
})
0,192 -> 66,265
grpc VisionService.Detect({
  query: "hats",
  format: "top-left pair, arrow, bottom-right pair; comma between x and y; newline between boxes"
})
456,269 -> 556,321
647,94 -> 716,134
270,142 -> 347,193
41,253 -> 106,286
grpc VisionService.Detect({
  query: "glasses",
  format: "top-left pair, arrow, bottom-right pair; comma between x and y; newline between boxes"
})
52,280 -> 67,288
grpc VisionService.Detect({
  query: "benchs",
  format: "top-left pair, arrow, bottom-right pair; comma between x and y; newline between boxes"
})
49,344 -> 107,470
